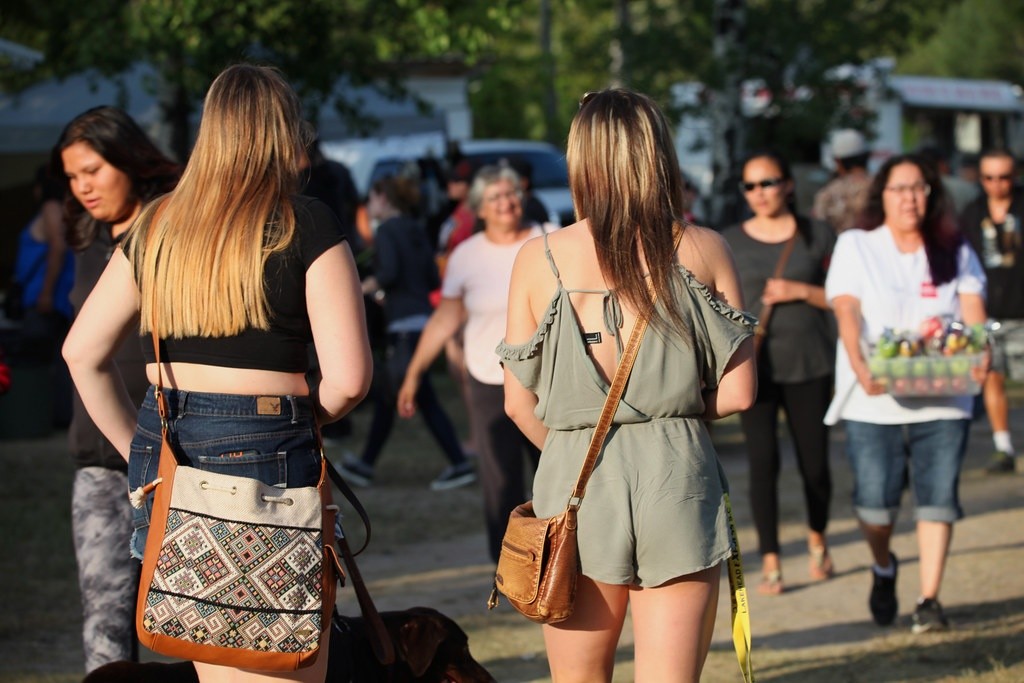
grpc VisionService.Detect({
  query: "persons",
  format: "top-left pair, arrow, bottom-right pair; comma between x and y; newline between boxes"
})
60,65 -> 375,683
54,104 -> 183,674
496,88 -> 759,683
15,149 -> 1024,633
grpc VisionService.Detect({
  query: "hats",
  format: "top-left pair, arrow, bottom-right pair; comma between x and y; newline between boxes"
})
831,129 -> 869,159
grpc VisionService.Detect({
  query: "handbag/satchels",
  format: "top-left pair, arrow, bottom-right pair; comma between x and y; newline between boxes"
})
487,501 -> 580,625
81,540 -> 497,683
136,430 -> 347,674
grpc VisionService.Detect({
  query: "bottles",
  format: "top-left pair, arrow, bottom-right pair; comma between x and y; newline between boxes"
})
866,316 -> 994,398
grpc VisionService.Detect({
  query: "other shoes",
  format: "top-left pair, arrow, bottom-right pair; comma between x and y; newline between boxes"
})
808,544 -> 833,581
984,451 -> 1015,472
759,569 -> 783,597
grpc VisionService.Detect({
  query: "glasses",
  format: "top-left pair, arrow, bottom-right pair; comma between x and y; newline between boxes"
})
743,178 -> 785,191
885,182 -> 928,195
984,175 -> 1010,181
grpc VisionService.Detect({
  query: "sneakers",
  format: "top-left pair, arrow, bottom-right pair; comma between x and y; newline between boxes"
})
911,597 -> 949,634
333,461 -> 370,487
430,463 -> 478,491
870,552 -> 899,626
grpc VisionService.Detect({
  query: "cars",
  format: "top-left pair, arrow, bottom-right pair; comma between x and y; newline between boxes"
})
351,135 -> 576,230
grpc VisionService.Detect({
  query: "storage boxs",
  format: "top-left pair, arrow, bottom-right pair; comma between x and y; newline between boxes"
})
867,342 -> 987,396
